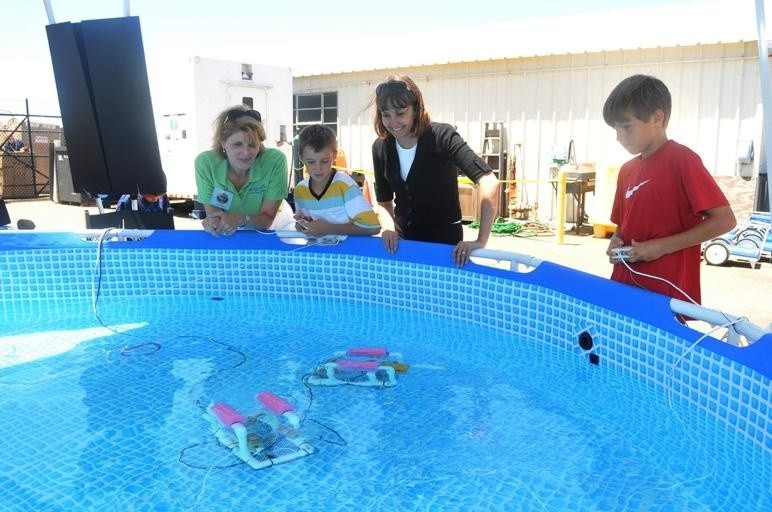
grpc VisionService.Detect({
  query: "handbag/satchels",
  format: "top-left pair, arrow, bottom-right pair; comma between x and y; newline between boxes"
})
238,213 -> 250,227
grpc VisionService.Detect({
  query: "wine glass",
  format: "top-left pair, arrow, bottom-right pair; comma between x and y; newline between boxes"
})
461,251 -> 465,255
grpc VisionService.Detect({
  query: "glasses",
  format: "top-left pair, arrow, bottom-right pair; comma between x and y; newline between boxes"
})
736,138 -> 754,180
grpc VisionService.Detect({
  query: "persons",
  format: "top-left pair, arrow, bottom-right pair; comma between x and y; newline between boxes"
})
115,169 -> 172,213
602,73 -> 737,307
291,124 -> 381,237
4,135 -> 24,152
372,73 -> 501,268
195,103 -> 289,237
276,139 -> 296,189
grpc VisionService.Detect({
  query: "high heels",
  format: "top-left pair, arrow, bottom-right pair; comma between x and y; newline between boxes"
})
361,178 -> 375,208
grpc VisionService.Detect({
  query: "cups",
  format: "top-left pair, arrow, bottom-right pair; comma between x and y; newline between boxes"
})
223,109 -> 262,125
